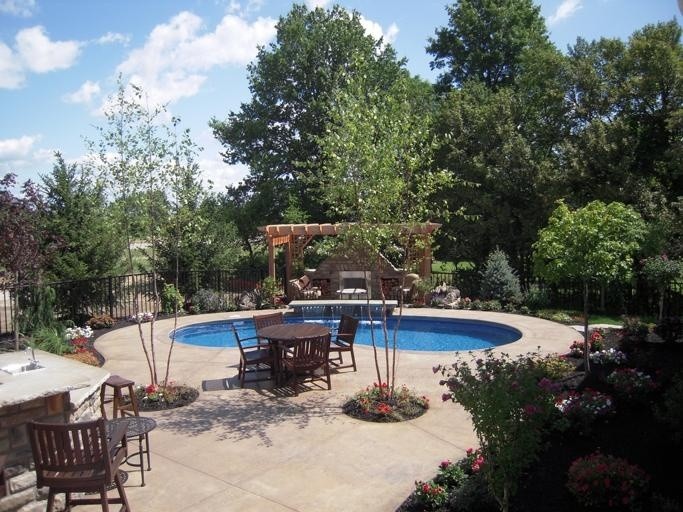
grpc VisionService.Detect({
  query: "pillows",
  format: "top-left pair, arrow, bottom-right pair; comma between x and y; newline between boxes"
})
299,275 -> 312,290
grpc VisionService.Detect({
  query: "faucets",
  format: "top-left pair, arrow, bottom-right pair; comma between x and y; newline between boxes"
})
24,345 -> 39,365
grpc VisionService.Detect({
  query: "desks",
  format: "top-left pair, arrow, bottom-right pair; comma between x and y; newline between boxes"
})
93,418 -> 156,486
256,324 -> 331,378
336,287 -> 367,299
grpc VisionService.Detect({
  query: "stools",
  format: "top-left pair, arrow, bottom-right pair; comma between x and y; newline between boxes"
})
100,376 -> 138,418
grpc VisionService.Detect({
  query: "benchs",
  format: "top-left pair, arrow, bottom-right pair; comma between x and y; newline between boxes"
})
288,298 -> 398,315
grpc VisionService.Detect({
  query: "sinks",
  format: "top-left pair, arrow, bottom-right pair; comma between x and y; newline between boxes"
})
0,360 -> 50,377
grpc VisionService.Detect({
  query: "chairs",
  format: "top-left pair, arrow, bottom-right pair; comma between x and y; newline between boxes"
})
280,333 -> 330,397
319,315 -> 359,373
252,312 -> 298,367
289,277 -> 321,300
231,321 -> 276,388
27,420 -> 137,512
390,273 -> 420,304
